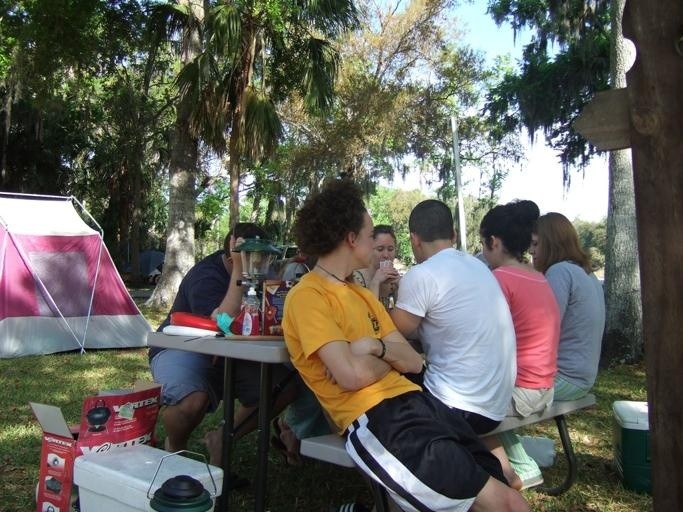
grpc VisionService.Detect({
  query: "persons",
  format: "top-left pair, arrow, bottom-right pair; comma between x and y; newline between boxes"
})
272,255 -> 365,471
391,199 -> 524,488
358,225 -> 401,300
497,213 -> 606,487
481,199 -> 559,415
282,187 -> 530,508
148,225 -> 272,470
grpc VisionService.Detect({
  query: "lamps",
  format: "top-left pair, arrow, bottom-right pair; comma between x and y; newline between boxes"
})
233,235 -> 280,315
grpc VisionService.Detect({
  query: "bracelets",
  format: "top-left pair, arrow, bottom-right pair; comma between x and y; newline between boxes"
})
376,338 -> 387,359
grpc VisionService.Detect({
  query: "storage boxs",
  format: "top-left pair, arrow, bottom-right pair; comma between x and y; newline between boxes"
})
25,382 -> 166,511
71,441 -> 224,511
609,399 -> 651,497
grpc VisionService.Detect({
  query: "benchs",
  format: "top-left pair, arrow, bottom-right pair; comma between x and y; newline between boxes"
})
297,391 -> 598,510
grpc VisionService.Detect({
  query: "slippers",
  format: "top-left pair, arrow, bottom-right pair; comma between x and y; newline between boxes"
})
334,502 -> 369,511
272,418 -> 285,439
270,437 -> 305,471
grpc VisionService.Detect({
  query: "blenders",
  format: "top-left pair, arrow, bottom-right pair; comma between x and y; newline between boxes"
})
231,235 -> 281,336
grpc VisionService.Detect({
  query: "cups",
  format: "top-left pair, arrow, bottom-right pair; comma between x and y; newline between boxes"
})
380,261 -> 393,283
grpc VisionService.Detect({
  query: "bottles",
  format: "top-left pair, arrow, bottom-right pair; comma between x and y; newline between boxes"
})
241,287 -> 259,336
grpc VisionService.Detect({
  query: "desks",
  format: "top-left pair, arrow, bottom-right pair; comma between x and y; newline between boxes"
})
145,331 -> 291,511
